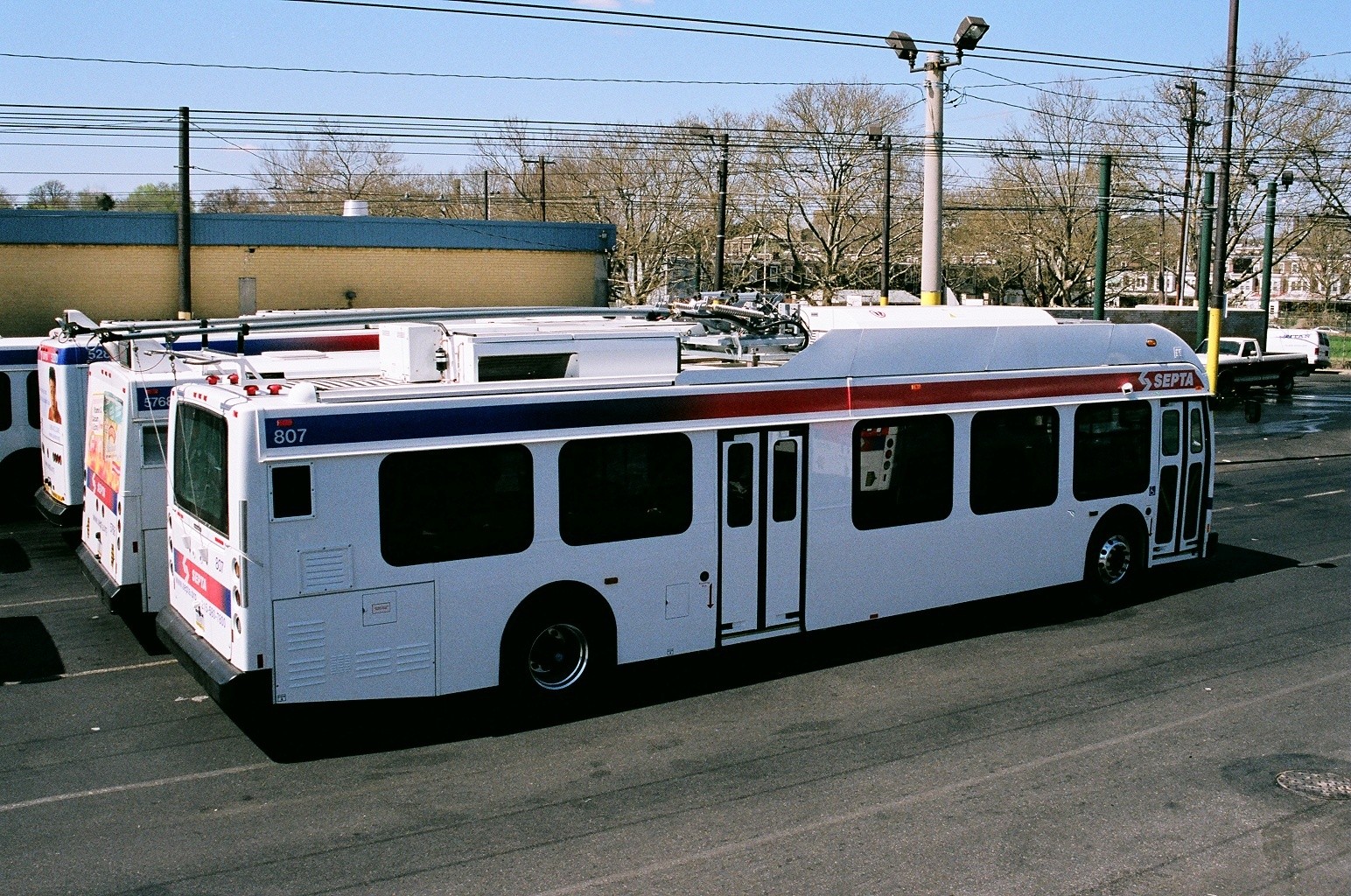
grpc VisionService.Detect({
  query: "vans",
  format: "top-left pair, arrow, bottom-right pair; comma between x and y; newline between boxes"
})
1266,327 -> 1331,376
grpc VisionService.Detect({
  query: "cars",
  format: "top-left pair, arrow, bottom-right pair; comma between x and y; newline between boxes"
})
1312,326 -> 1345,335
1268,323 -> 1287,329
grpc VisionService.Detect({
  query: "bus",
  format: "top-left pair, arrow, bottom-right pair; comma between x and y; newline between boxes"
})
154,276 -> 1217,721
0,290 -> 1119,633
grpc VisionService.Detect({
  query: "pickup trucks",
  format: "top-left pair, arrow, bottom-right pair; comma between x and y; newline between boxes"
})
1191,337 -> 1310,397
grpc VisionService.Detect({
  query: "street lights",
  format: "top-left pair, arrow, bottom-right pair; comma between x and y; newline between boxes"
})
866,126 -> 892,306
885,16 -> 991,305
690,122 -> 730,293
1246,170 -> 1295,350
